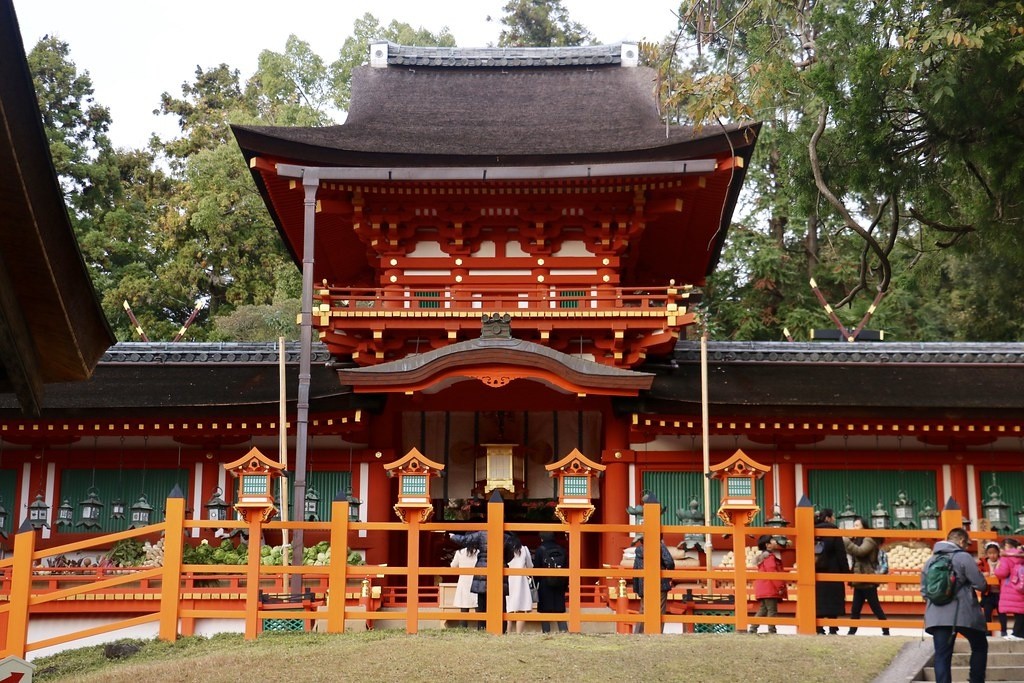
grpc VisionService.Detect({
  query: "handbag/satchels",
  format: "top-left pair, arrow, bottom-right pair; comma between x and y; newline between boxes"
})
528,574 -> 540,603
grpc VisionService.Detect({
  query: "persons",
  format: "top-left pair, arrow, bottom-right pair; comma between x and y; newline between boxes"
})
448,530 -> 534,634
813,509 -> 853,635
529,531 -> 569,633
749,534 -> 789,633
918,528 -> 989,683
632,532 -> 676,635
841,518 -> 890,636
976,538 -> 1024,642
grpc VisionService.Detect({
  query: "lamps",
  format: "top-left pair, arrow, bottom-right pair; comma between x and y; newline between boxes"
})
0,431 -> 363,548
625,429 -> 1024,554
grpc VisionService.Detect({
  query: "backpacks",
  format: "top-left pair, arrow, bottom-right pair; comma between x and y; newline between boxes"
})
1010,564 -> 1023,592
814,540 -> 826,563
923,554 -> 958,605
542,549 -> 569,593
873,549 -> 888,574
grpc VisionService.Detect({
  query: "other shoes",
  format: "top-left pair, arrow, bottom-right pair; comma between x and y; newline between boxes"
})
1002,633 -> 1024,640
